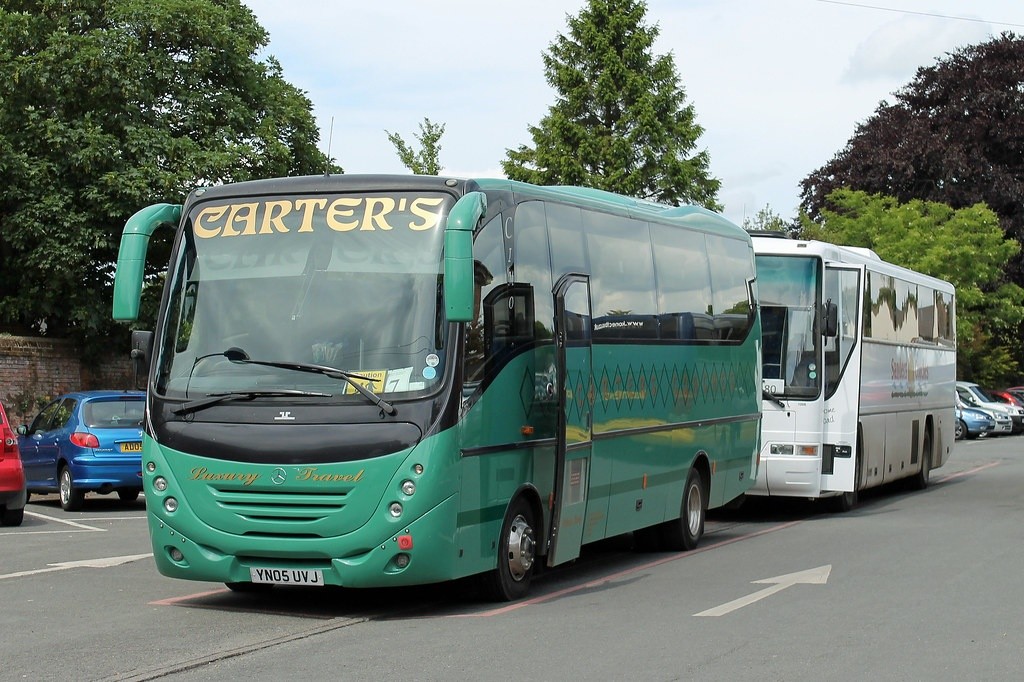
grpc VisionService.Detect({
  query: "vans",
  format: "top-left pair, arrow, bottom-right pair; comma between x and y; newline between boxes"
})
956,380 -> 1014,437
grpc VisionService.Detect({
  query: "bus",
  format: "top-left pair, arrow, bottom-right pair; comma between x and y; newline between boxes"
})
746,230 -> 958,511
109,174 -> 770,600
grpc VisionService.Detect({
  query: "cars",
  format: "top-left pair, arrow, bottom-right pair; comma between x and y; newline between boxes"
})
13,389 -> 153,515
954,391 -> 995,442
984,387 -> 1024,435
0,401 -> 27,527
